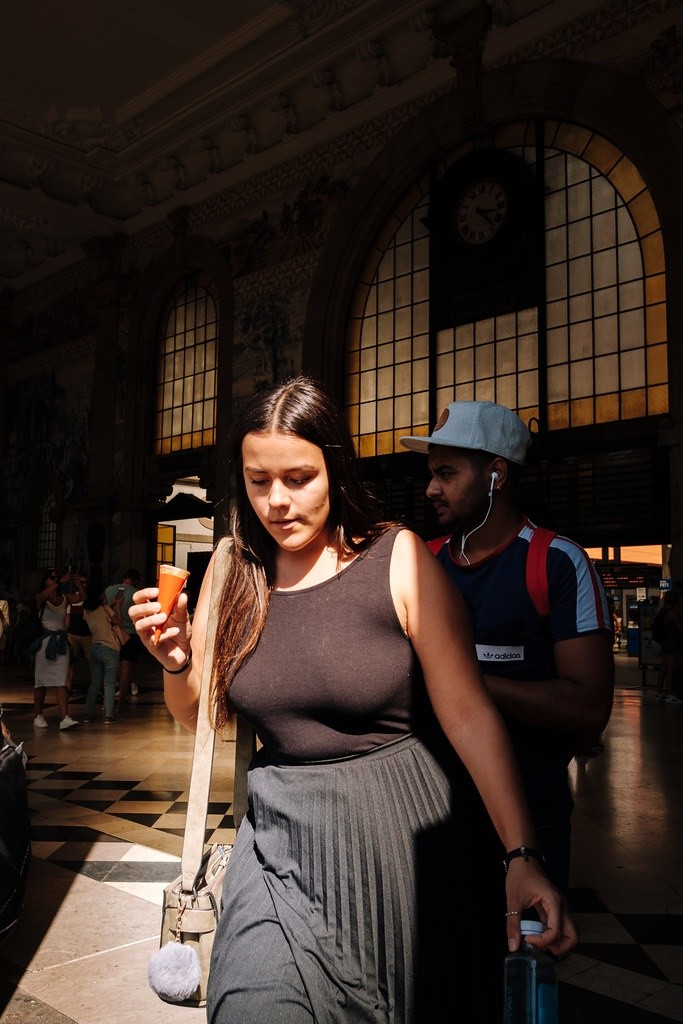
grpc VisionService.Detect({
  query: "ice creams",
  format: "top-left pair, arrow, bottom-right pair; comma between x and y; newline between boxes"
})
160,564 -> 190,581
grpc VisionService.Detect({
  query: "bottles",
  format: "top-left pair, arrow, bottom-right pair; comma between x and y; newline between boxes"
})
503,920 -> 557,1024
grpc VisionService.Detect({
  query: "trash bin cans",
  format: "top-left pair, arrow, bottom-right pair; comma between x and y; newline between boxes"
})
627,628 -> 640,656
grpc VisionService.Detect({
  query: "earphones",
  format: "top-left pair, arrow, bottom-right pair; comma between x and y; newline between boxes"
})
491,472 -> 498,489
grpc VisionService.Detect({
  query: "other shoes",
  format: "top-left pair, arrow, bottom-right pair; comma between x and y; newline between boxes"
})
82,715 -> 99,723
104,717 -> 120,724
131,681 -> 139,696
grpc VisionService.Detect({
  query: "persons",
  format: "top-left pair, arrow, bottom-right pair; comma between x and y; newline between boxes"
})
0,594 -> 42,667
650,580 -> 683,704
128,380 -> 580,1023
25,568 -> 140,730
400,399 -> 616,1024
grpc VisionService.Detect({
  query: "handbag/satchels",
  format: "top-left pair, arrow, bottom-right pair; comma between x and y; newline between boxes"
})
101,606 -> 131,646
24,599 -> 48,637
147,843 -> 239,1010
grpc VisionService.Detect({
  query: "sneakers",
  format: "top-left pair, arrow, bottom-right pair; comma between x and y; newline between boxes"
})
33,714 -> 48,728
59,716 -> 79,730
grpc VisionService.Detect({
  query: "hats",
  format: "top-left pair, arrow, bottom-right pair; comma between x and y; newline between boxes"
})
399,399 -> 531,465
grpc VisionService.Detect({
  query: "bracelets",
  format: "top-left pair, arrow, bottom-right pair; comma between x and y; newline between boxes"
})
503,845 -> 546,873
159,650 -> 192,674
54,580 -> 64,587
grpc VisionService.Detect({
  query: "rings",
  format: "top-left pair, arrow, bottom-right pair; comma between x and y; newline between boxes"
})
505,911 -> 522,917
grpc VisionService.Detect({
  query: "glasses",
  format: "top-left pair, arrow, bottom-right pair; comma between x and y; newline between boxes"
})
48,576 -> 61,581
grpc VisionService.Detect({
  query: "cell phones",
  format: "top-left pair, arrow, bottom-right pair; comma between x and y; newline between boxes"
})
69,566 -> 72,572
116,587 -> 125,600
